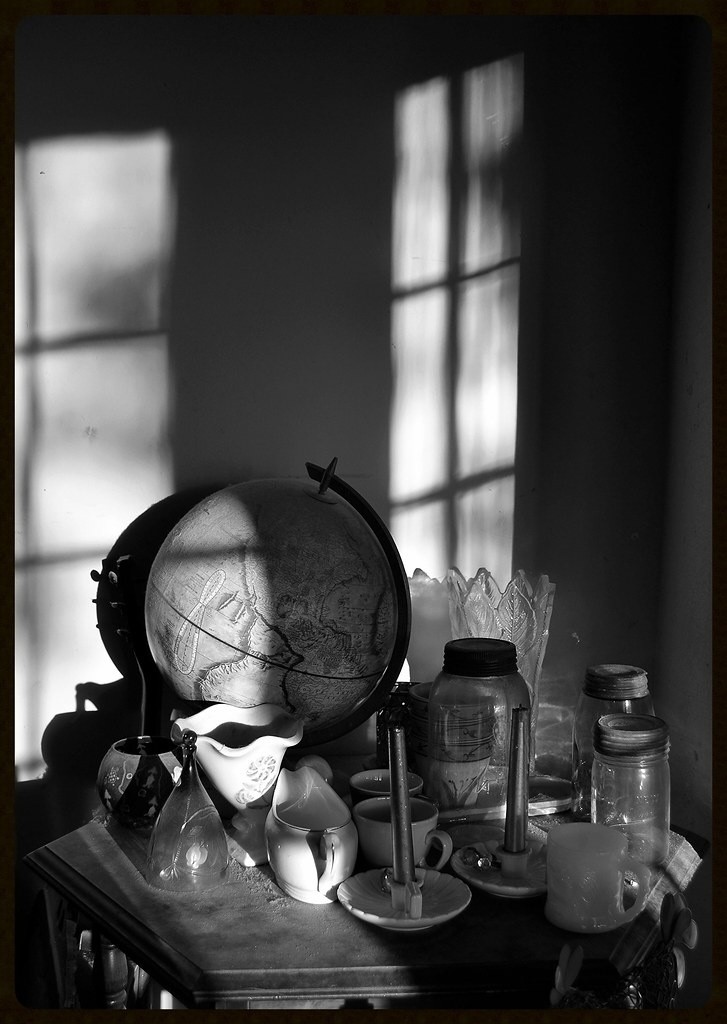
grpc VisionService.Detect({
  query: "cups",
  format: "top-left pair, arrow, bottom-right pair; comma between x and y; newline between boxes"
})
409,682 -> 495,809
572,665 -> 655,817
591,713 -> 671,865
446,567 -> 555,773
349,769 -> 440,808
545,823 -> 650,934
355,797 -> 452,870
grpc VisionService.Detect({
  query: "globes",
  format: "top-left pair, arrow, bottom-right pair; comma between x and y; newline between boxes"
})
145,458 -> 413,770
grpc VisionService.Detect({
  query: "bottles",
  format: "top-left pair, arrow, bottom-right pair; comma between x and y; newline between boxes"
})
426,638 -> 530,808
146,733 -> 229,892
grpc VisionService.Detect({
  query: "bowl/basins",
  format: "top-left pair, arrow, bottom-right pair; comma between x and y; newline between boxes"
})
97,737 -> 182,831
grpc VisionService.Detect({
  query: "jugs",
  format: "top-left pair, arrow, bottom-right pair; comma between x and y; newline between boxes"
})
264,766 -> 358,906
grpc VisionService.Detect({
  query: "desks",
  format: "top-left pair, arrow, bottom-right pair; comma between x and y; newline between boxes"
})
25,757 -> 711,1010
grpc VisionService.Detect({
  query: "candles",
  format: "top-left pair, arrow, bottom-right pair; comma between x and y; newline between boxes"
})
504,704 -> 530,852
388,724 -> 416,882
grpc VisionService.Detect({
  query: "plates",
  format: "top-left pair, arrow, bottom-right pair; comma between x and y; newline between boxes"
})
437,776 -> 574,823
